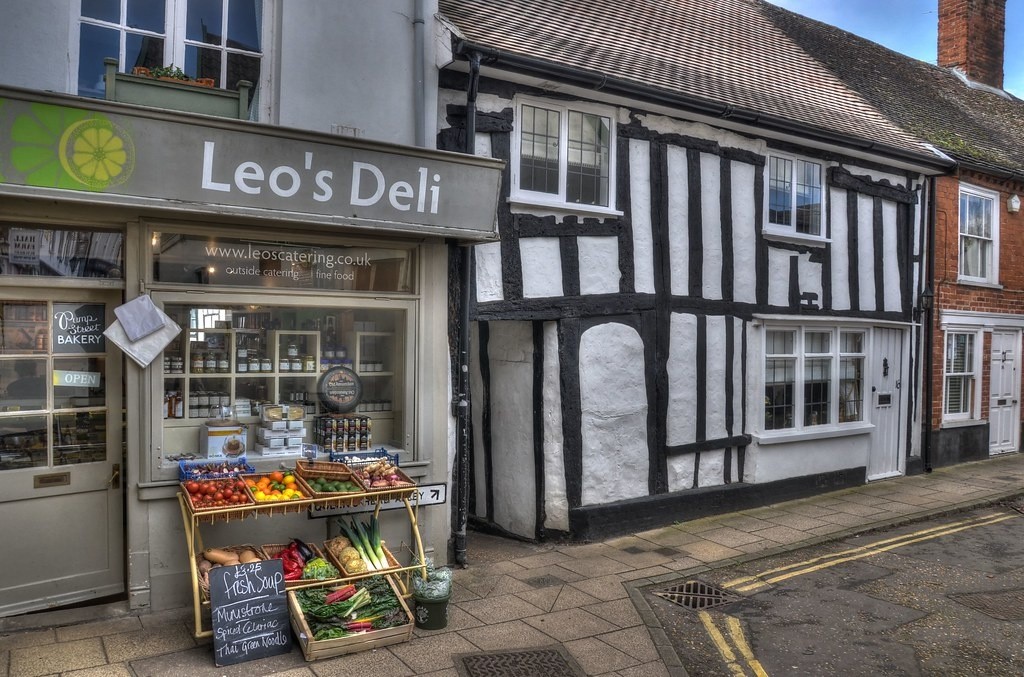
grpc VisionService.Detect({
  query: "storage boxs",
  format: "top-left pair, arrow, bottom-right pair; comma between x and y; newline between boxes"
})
255,420 -> 306,456
288,575 -> 415,661
329,447 -> 399,470
354,321 -> 375,355
199,423 -> 247,459
235,399 -> 251,418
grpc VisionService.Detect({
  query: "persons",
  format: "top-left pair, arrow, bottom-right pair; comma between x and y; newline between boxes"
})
5,359 -> 46,397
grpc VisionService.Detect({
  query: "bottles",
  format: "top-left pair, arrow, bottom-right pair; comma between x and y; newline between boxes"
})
163,391 -> 184,420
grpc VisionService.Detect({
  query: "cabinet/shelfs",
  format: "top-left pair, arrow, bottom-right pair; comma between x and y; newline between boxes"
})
177,492 -> 427,637
162,329 -> 394,453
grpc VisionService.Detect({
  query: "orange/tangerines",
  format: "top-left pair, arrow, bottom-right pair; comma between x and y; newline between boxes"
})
245,472 -> 298,495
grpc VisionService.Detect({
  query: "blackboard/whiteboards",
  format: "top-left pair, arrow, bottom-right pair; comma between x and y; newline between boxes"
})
205,557 -> 293,667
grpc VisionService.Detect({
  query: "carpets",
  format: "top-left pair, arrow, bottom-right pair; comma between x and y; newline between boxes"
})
451,643 -> 590,677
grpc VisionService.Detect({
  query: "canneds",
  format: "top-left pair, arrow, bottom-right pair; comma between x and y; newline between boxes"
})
158,343 -> 392,421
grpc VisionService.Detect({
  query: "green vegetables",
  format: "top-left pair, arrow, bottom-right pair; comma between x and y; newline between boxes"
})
294,575 -> 409,641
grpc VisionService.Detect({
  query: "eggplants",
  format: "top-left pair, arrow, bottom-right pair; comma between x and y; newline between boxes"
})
287,536 -> 313,562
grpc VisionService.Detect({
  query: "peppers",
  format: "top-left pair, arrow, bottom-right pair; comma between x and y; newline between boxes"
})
273,542 -> 307,580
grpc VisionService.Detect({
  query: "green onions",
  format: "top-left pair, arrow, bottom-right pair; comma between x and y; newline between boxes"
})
334,513 -> 389,572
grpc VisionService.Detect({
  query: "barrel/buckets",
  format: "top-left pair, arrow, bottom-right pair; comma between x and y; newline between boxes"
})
413,594 -> 449,629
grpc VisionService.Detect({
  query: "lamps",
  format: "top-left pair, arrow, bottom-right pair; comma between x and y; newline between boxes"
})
914,285 -> 934,320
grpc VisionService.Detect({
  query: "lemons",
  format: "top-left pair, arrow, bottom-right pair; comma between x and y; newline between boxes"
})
253,475 -> 304,501
